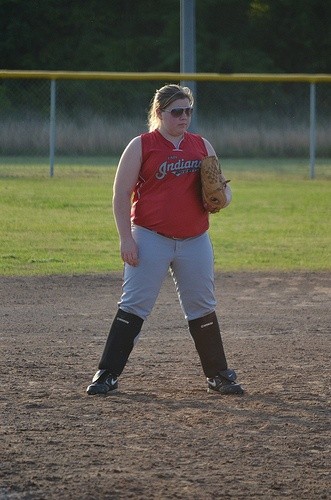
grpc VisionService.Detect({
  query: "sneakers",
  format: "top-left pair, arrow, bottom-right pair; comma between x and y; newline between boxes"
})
86,369 -> 119,395
206,369 -> 244,397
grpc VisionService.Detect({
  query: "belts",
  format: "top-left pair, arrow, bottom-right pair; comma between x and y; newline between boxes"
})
145,228 -> 193,241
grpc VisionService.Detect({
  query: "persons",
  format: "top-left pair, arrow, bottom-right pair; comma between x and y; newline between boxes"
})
85,85 -> 244,397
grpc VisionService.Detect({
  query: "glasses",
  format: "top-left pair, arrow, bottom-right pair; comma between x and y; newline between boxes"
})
162,107 -> 193,118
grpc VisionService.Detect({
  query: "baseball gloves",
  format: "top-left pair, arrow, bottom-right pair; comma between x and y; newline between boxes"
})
199,155 -> 230,212
